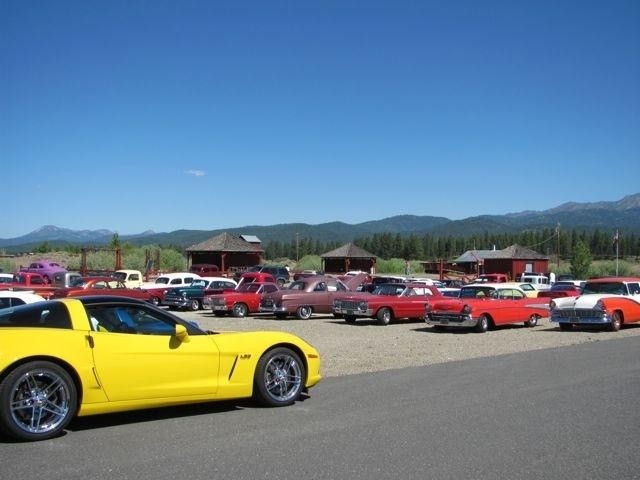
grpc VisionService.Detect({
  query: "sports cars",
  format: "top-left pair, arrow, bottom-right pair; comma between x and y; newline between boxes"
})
0,295 -> 322,441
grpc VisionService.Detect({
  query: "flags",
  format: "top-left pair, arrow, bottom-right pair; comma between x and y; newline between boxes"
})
611,228 -> 619,249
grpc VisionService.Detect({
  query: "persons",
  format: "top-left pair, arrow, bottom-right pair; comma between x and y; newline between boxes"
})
403,260 -> 411,276
